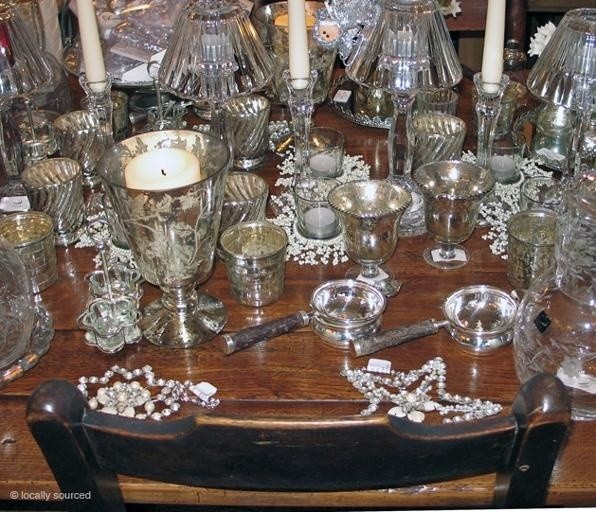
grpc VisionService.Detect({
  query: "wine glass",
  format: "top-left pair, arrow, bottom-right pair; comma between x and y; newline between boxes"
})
0,1 -> 596,422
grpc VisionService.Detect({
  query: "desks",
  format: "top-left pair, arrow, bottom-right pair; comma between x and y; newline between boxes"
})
1,145 -> 595,512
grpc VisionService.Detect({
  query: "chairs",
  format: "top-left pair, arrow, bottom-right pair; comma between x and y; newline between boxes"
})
23,372 -> 575,510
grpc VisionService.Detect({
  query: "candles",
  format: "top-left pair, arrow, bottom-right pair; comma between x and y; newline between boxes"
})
286,0 -> 312,92
479,0 -> 507,95
124,147 -> 201,192
75,0 -> 109,96
